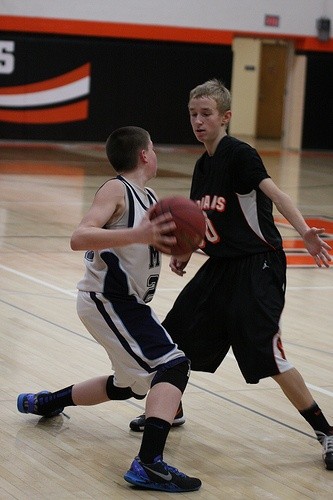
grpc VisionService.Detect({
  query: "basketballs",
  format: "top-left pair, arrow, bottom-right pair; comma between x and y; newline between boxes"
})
150,196 -> 206,256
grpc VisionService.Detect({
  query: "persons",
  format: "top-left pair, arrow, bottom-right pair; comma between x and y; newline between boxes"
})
129,79 -> 333,472
16,124 -> 202,492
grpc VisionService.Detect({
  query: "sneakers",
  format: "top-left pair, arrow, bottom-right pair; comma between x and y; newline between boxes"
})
317,426 -> 333,470
124,456 -> 202,492
17,391 -> 64,416
129,400 -> 186,431
